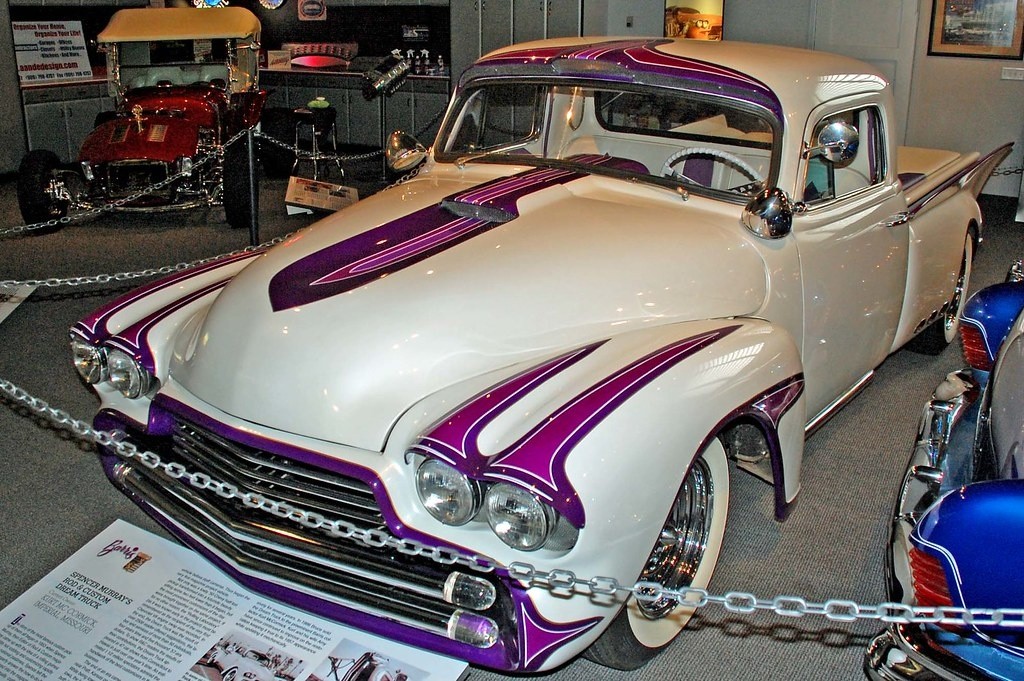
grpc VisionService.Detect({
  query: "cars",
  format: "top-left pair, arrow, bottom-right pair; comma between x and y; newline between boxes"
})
860,255 -> 1024,681
67,34 -> 1018,678
15,4 -> 268,238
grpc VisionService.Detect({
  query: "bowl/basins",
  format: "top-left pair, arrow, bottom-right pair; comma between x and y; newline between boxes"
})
307,101 -> 329,108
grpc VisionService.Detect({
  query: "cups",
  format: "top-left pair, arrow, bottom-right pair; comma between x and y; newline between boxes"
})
316,96 -> 326,101
428,69 -> 435,75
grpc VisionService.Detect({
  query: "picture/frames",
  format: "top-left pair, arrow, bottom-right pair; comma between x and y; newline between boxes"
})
927,0 -> 1024,60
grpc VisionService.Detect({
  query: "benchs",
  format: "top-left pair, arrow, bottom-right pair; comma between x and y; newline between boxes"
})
556,137 -> 871,208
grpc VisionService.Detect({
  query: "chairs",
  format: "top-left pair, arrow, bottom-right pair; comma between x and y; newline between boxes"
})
199,65 -> 228,82
145,66 -> 184,86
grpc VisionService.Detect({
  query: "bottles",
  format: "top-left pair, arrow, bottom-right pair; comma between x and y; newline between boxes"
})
438,55 -> 444,76
415,55 -> 421,74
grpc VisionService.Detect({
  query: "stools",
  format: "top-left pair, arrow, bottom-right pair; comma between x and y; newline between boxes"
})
292,108 -> 345,185
